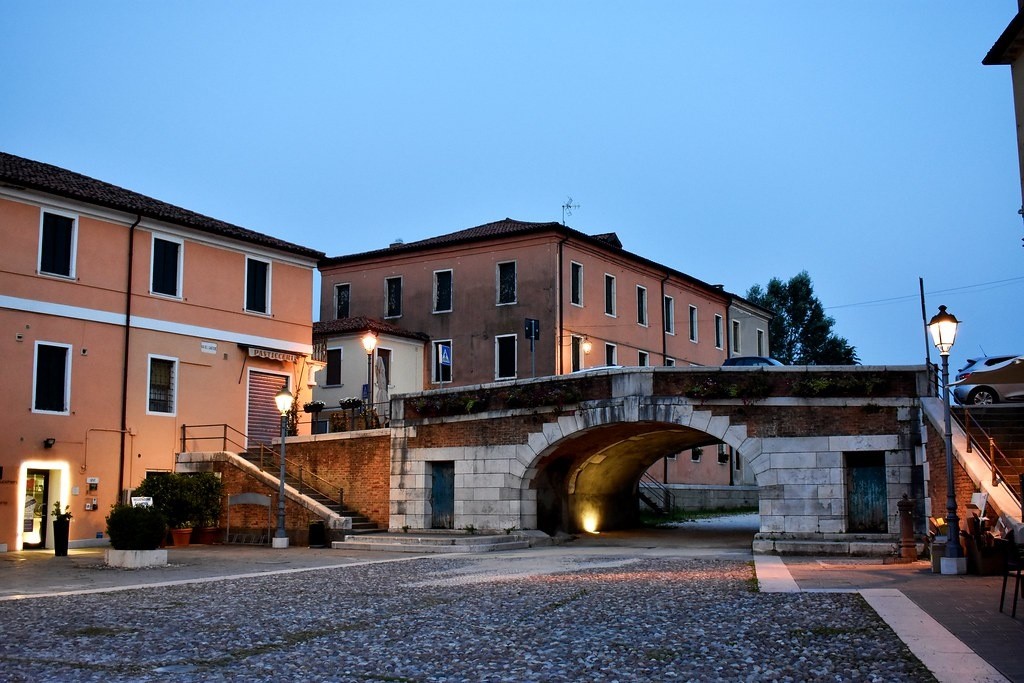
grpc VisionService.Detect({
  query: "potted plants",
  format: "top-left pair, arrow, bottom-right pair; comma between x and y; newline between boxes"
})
197,519 -> 218,544
171,519 -> 193,546
51,501 -> 74,557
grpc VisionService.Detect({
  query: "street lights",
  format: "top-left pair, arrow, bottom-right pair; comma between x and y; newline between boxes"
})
273,383 -> 294,537
924,304 -> 966,558
359,333 -> 377,429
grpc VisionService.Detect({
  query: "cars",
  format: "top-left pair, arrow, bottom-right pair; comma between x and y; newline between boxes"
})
722,355 -> 785,367
952,353 -> 1024,406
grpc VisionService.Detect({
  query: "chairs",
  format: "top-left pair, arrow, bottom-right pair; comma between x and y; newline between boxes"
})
999,543 -> 1024,618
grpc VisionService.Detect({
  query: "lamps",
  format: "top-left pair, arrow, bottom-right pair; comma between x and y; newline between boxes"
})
43,438 -> 55,448
561,334 -> 593,355
